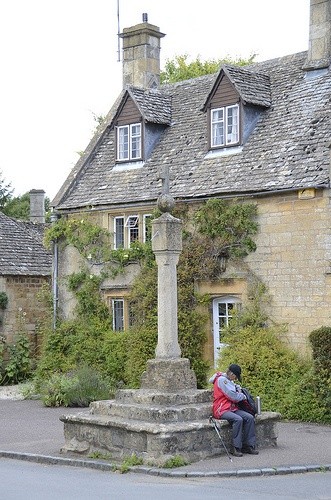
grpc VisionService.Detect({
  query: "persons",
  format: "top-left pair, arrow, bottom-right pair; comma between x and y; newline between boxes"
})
209,364 -> 260,457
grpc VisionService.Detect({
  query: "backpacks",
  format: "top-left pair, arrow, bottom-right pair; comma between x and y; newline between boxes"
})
238,388 -> 257,416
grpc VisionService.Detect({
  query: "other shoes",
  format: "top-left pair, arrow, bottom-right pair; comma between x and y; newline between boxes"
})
243,446 -> 259,454
229,446 -> 242,457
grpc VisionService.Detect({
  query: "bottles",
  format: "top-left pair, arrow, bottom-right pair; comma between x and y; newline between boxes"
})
256,396 -> 261,415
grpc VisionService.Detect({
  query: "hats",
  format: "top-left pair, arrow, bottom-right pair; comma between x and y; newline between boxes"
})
229,364 -> 242,382
